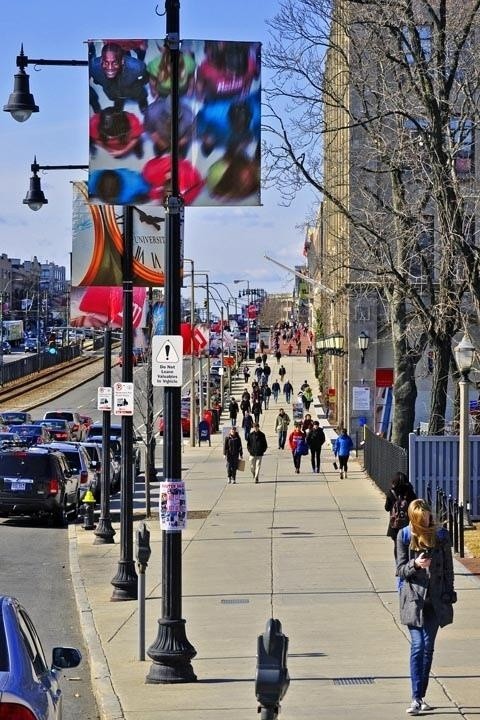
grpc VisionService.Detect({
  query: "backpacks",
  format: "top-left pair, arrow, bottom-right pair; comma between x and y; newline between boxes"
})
390,489 -> 409,529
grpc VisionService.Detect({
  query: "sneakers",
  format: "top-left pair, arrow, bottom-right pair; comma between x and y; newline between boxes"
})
406,700 -> 431,713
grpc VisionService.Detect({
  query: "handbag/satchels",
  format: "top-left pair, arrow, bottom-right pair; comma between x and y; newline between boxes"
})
237,460 -> 245,471
333,462 -> 338,469
296,439 -> 308,455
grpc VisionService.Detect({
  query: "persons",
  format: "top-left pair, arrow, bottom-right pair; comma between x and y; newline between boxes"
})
211,319 -> 353,484
396,498 -> 457,717
89,40 -> 260,206
384,471 -> 419,558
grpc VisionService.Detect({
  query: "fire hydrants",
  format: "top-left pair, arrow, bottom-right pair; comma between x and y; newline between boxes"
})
80,490 -> 97,529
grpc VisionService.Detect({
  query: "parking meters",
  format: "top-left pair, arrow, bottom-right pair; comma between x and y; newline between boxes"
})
136,523 -> 152,663
253,617 -> 290,720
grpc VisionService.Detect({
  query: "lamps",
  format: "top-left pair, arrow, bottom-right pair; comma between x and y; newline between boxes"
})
316,333 -> 347,357
358,331 -> 369,361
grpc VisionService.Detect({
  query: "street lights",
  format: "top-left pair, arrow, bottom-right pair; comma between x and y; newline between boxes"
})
6,4 -> 203,684
183,274 -> 256,449
24,155 -> 136,606
1,278 -> 22,317
452,329 -> 477,533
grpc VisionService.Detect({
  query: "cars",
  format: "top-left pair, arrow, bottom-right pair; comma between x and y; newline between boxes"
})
1,596 -> 89,720
0,408 -> 143,525
160,318 -> 255,441
1,319 -> 146,369
